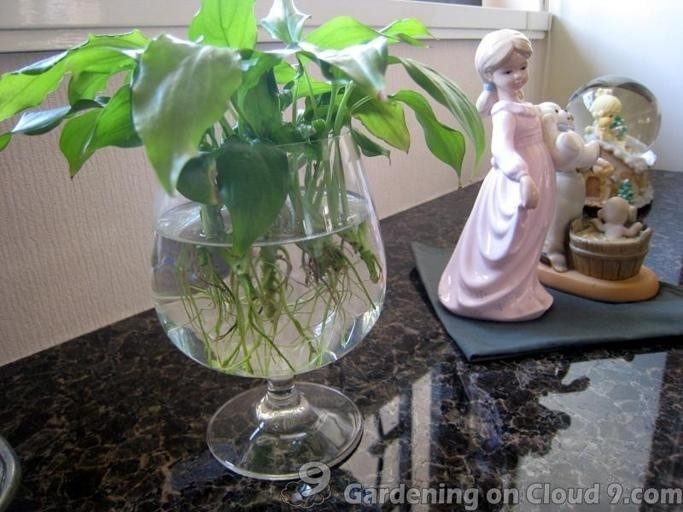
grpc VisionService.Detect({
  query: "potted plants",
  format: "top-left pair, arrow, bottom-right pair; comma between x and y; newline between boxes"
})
0,0 -> 486,478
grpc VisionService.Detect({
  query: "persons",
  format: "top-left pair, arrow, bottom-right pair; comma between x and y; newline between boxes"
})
438,27 -> 662,325
